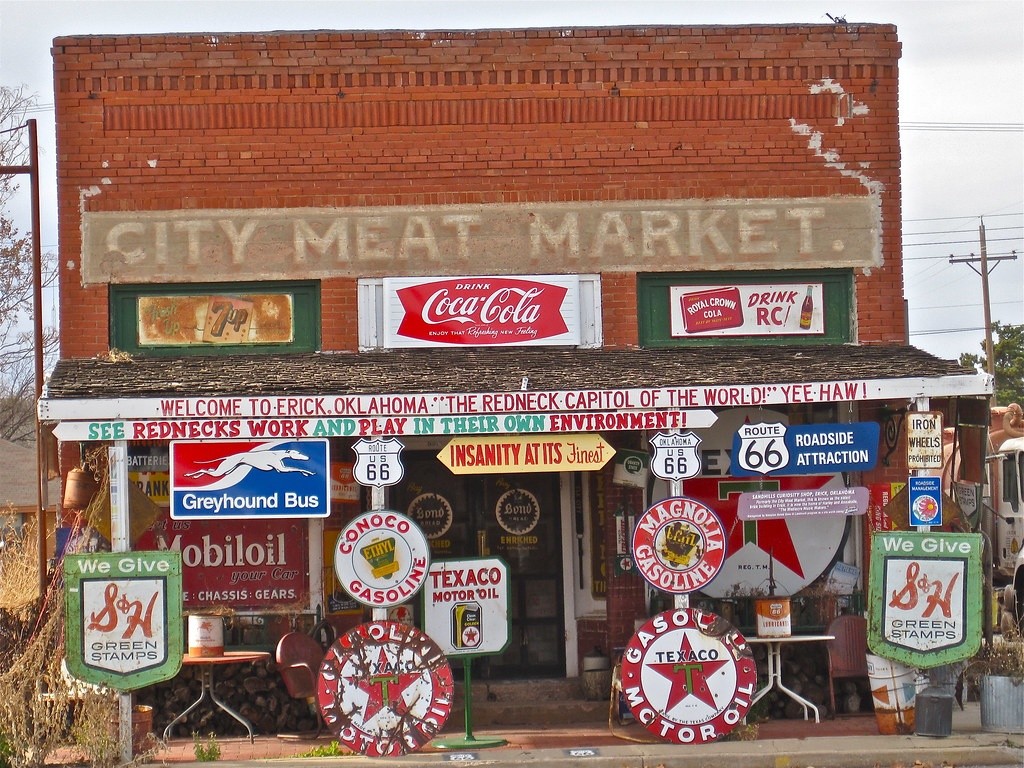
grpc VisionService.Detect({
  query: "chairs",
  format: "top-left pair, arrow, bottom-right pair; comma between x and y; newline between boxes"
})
277,632 -> 325,739
826,616 -> 867,712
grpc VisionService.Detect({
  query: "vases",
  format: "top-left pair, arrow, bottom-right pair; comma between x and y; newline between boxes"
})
980,676 -> 1024,734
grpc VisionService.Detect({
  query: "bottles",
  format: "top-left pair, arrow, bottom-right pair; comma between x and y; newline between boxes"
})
800,287 -> 814,330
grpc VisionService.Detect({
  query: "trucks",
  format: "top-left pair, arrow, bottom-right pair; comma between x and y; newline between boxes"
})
931,407 -> 1024,630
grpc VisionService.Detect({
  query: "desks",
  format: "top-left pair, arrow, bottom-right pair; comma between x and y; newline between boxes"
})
164,651 -> 272,744
742,635 -> 835,725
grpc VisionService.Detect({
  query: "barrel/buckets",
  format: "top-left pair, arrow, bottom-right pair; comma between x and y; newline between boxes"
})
753,579 -> 792,637
187,614 -> 224,657
